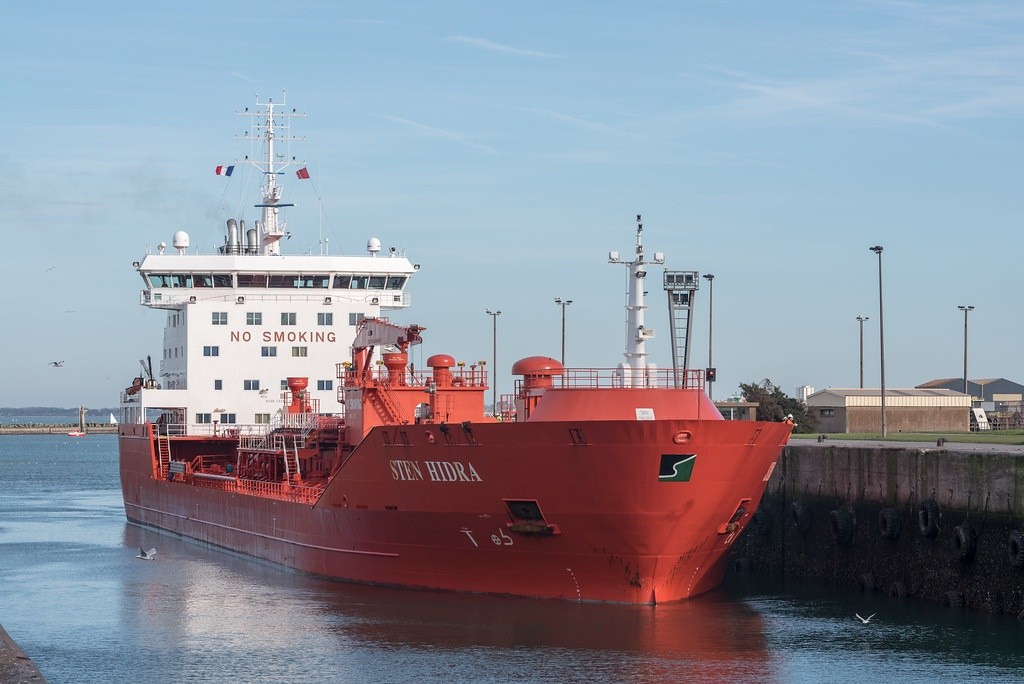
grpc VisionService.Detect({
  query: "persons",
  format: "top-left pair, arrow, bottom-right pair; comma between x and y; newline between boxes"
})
782,413 -> 797,444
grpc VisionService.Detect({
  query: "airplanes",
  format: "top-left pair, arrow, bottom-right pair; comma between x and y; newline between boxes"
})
111,209 -> 798,611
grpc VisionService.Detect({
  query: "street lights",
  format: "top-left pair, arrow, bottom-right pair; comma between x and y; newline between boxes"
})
870,245 -> 888,439
856,315 -> 869,389
958,305 -> 975,395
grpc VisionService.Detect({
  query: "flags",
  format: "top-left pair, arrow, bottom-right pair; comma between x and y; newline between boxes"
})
214,166 -> 235,176
295,168 -> 310,180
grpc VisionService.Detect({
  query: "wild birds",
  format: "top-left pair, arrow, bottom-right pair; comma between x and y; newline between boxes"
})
856,613 -> 876,624
48,361 -> 64,368
135,545 -> 157,560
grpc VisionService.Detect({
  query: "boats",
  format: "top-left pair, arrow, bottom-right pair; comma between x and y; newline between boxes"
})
68,431 -> 85,436
